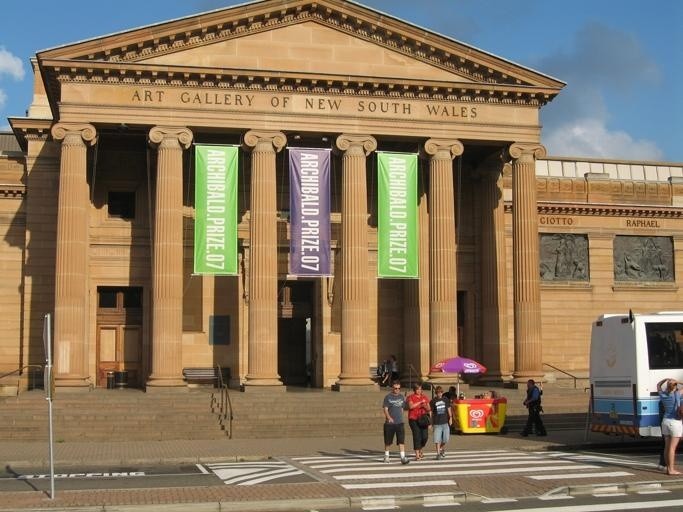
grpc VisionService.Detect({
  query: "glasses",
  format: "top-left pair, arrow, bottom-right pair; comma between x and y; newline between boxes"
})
395,387 -> 401,390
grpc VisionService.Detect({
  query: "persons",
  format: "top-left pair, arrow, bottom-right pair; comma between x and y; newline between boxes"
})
443,386 -> 456,399
390,355 -> 400,381
405,382 -> 431,461
379,359 -> 390,385
657,378 -> 683,475
429,385 -> 453,460
519,379 -> 547,437
382,379 -> 410,465
657,400 -> 668,473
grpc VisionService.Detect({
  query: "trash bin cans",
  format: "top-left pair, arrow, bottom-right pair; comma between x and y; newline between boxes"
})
107,371 -> 129,389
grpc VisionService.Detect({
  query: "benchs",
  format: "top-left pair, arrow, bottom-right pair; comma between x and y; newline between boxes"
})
182,366 -> 233,388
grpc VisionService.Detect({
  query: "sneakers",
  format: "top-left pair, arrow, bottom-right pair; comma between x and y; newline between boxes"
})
401,457 -> 410,464
658,465 -> 667,470
416,449 -> 446,461
383,456 -> 390,463
537,432 -> 548,436
521,432 -> 529,437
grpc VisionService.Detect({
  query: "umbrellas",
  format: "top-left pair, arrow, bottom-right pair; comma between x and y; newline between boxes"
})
431,356 -> 487,400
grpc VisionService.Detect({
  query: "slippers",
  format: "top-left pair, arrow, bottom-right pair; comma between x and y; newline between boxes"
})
668,471 -> 682,475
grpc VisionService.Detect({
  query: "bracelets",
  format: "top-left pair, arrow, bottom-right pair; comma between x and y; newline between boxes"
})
449,416 -> 452,417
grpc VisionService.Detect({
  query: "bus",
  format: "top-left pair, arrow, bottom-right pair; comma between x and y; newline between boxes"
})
589,310 -> 683,438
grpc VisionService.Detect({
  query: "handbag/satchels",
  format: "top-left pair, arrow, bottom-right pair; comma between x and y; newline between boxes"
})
417,414 -> 431,428
676,406 -> 683,420
